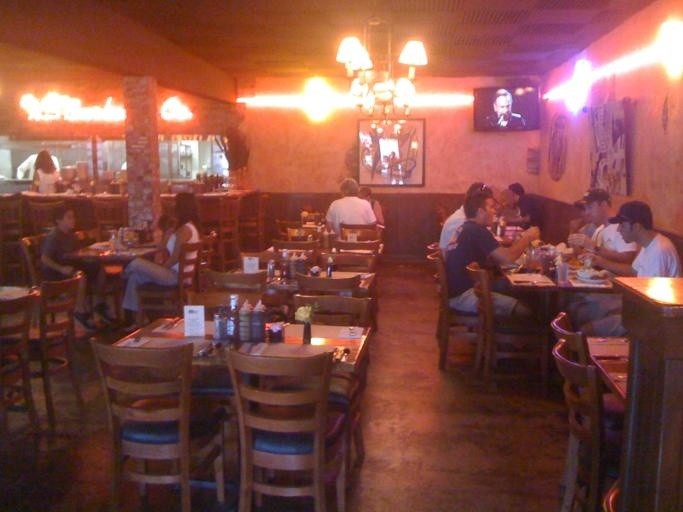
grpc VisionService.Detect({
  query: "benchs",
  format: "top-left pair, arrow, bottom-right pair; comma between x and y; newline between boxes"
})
536,196 -> 683,252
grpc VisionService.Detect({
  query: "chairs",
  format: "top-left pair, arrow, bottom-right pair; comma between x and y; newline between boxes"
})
550,310 -> 589,364
26,201 -> 64,224
20,234 -> 47,282
424,196 -> 536,252
427,250 -> 475,370
187,290 -> 284,315
90,338 -> 227,511
543,338 -> 621,510
0,200 -> 25,280
291,295 -> 373,326
0,288 -> 40,441
201,192 -> 384,293
35,269 -> 85,426
92,199 -> 128,224
225,350 -> 334,510
465,260 -> 548,380
134,242 -> 204,322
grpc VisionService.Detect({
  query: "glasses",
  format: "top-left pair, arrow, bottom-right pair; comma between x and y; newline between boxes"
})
479,183 -> 487,191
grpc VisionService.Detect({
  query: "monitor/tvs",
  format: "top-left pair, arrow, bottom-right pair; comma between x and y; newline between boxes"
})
473,84 -> 541,131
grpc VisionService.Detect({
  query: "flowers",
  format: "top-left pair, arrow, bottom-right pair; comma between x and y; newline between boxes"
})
292,300 -> 321,324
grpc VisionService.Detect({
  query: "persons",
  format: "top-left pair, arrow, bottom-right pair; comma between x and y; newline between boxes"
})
569,200 -> 680,340
483,89 -> 527,130
565,200 -> 597,249
444,190 -> 551,366
437,181 -> 505,278
357,186 -> 387,253
324,177 -> 383,254
30,149 -> 62,194
38,202 -> 116,332
496,180 -> 543,230
567,186 -> 639,329
115,190 -> 202,335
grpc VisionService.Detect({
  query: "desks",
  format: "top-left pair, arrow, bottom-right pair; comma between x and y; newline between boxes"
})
610,276 -> 682,511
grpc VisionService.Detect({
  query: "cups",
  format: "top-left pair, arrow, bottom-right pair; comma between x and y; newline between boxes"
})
557,261 -> 568,284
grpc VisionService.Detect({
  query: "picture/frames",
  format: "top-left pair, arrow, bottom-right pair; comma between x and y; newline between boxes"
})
358,118 -> 426,188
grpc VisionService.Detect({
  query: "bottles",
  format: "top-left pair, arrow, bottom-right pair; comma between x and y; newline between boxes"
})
268,249 -> 308,279
212,294 -> 266,346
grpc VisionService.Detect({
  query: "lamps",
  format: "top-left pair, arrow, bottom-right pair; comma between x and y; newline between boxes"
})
335,16 -> 428,113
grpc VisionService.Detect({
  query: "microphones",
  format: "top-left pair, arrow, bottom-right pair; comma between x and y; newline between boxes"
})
499,110 -> 511,122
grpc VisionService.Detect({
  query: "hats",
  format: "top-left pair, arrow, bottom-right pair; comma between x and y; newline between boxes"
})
573,189 -> 610,206
607,200 -> 652,225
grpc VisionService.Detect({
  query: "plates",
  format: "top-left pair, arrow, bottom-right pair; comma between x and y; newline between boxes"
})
574,273 -> 610,283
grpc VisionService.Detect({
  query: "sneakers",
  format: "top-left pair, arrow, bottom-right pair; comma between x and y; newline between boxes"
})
74,311 -> 96,330
94,302 -> 113,322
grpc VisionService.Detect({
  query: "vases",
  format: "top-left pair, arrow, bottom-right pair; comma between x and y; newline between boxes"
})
301,321 -> 312,346
